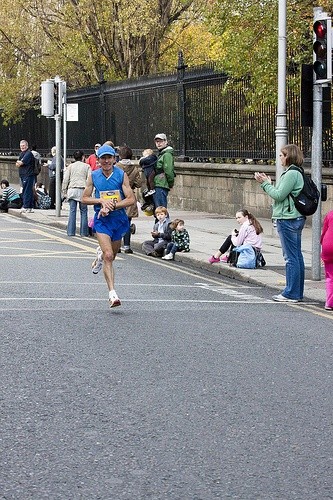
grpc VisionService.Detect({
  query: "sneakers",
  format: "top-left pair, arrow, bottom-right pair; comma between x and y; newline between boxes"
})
108,290 -> 121,308
161,252 -> 174,259
91,246 -> 104,274
272,294 -> 301,303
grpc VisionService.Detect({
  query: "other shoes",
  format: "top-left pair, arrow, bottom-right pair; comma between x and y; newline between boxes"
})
27,208 -> 34,213
20,207 -> 27,213
124,249 -> 133,254
220,255 -> 228,262
151,249 -> 161,257
145,190 -> 155,197
208,255 -> 220,263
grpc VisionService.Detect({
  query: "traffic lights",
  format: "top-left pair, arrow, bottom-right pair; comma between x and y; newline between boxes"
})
313,19 -> 328,80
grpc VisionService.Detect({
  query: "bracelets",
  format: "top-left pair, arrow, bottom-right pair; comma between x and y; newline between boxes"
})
113,203 -> 116,210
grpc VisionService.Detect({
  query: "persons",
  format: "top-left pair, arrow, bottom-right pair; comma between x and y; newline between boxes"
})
80,145 -> 136,308
61,150 -> 92,237
0,133 -> 191,261
253,143 -> 320,303
15,137 -> 35,213
208,209 -> 266,269
319,209 -> 333,310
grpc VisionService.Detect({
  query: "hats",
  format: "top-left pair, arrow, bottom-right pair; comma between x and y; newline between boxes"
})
97,145 -> 115,159
154,133 -> 167,141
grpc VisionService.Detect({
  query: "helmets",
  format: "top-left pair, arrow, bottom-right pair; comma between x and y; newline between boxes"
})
141,201 -> 154,216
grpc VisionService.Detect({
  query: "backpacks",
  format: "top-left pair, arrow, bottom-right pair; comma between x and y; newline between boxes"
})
285,167 -> 320,216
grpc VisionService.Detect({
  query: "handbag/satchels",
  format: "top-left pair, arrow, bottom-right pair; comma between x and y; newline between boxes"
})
233,244 -> 256,268
